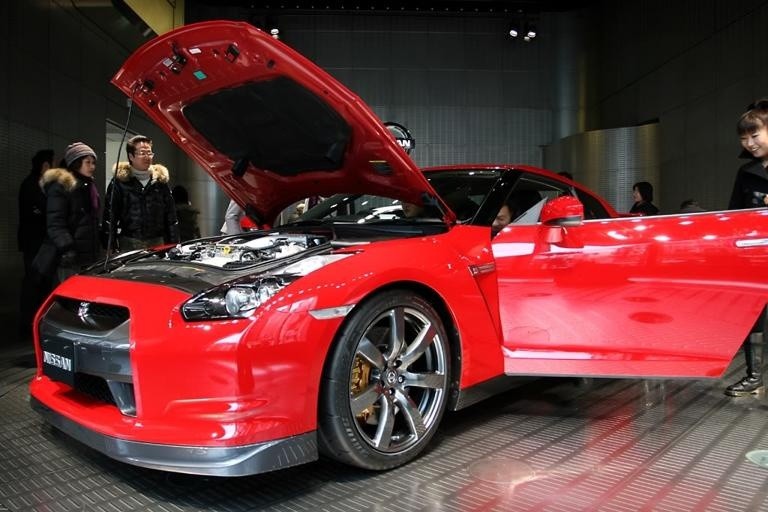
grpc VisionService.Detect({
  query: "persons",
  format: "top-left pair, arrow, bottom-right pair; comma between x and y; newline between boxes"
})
627,181 -> 660,213
16,133 -> 200,343
724,97 -> 768,399
289,204 -> 305,223
492,205 -> 512,236
679,198 -> 705,212
556,171 -> 573,179
218,198 -> 249,236
401,201 -> 425,219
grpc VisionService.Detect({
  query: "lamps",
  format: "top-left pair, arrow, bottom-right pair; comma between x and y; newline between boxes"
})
505,12 -> 540,43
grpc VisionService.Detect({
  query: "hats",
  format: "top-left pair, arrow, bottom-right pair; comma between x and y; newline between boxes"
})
63,141 -> 97,168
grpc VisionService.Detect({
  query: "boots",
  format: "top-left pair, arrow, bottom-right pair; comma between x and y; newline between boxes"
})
724,330 -> 765,398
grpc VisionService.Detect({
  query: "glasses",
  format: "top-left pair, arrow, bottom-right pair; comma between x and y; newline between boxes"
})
135,151 -> 154,159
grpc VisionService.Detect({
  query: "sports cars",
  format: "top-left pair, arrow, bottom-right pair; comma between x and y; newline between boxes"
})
27,19 -> 768,479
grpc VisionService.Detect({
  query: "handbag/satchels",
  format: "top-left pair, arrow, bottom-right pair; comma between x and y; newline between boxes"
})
31,238 -> 57,278
